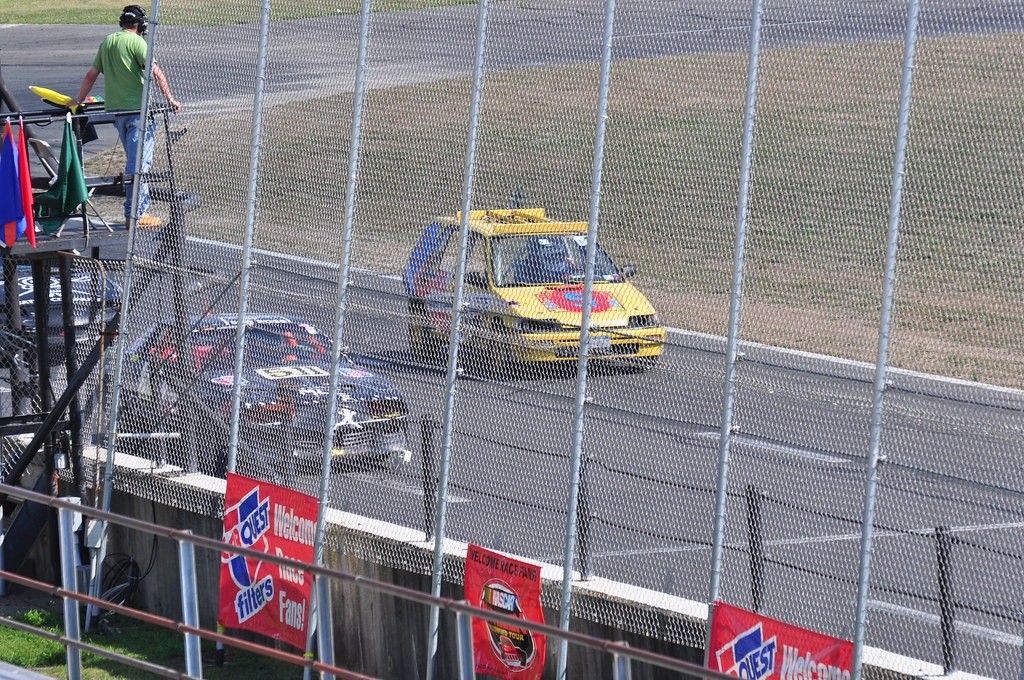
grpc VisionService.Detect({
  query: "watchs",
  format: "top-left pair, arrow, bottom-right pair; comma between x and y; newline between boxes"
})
76,98 -> 80,104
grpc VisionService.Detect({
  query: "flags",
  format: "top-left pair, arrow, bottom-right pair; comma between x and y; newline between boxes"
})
708,601 -> 854,679
463,544 -> 546,680
18,116 -> 37,249
218,473 -> 321,652
31,112 -> 89,236
0,116 -> 27,249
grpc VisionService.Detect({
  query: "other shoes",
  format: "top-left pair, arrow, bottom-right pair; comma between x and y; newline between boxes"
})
126,214 -> 161,228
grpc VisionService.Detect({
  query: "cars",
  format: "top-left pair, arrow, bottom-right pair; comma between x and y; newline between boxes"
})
98,304 -> 412,482
400,204 -> 664,380
0,255 -> 124,369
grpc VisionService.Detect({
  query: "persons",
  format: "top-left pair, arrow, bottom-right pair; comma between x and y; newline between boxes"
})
66,5 -> 181,230
532,242 -> 574,282
